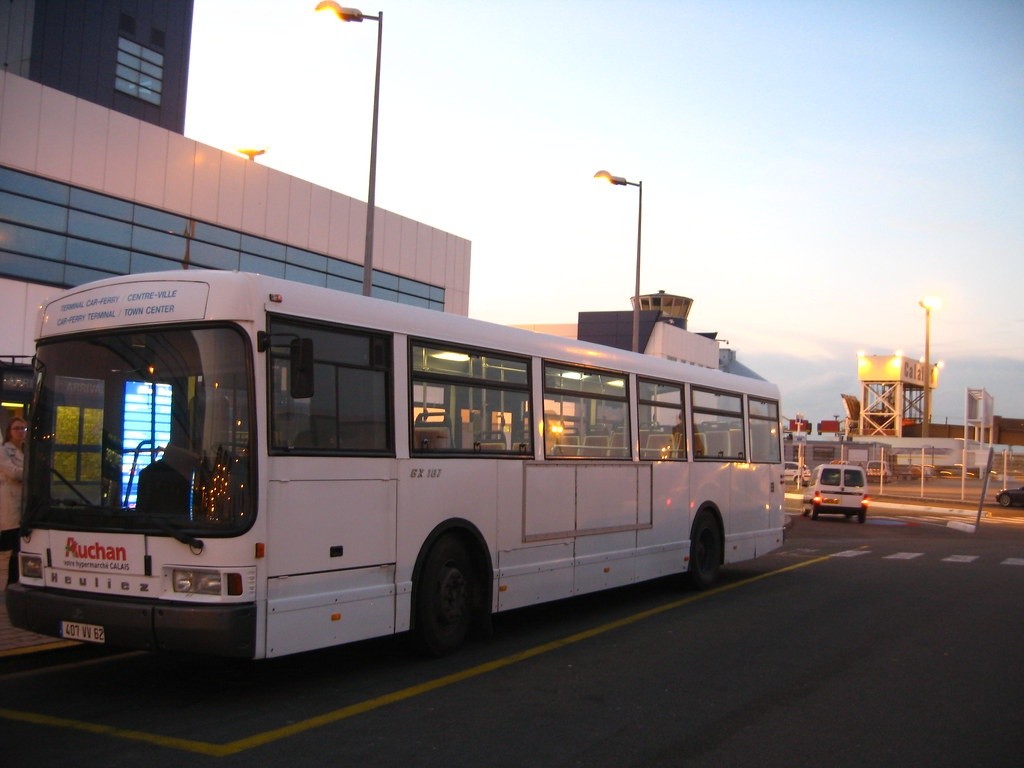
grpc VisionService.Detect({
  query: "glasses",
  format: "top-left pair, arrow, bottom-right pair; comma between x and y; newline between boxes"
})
9,426 -> 26,430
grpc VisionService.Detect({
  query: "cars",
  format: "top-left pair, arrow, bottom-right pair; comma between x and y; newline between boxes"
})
866,460 -> 892,483
784,461 -> 812,487
995,485 -> 1024,507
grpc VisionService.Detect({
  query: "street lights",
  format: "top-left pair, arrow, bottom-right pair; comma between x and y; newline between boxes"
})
314,0 -> 383,299
596,170 -> 643,352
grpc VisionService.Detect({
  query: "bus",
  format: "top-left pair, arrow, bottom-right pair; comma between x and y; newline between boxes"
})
0,267 -> 789,663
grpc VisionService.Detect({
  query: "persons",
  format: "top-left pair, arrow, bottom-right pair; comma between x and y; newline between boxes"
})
0,417 -> 28,592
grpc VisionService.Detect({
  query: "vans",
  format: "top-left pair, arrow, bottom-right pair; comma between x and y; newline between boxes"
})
800,463 -> 870,523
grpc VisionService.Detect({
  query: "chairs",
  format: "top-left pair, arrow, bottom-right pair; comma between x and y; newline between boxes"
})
413,419 -> 753,461
